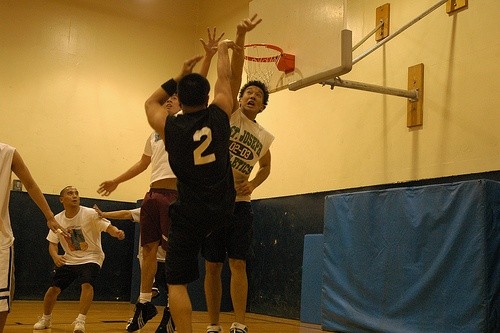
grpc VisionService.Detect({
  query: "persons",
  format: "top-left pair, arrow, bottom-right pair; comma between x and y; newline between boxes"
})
94,14 -> 275,333
0,142 -> 72,333
33,186 -> 125,333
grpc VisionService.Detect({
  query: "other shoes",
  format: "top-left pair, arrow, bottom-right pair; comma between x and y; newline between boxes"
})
230,322 -> 248,333
207,324 -> 222,333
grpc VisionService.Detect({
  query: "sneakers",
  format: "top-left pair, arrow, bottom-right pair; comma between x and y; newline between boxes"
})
72,319 -> 86,333
34,317 -> 52,329
154,307 -> 175,333
126,302 -> 159,333
